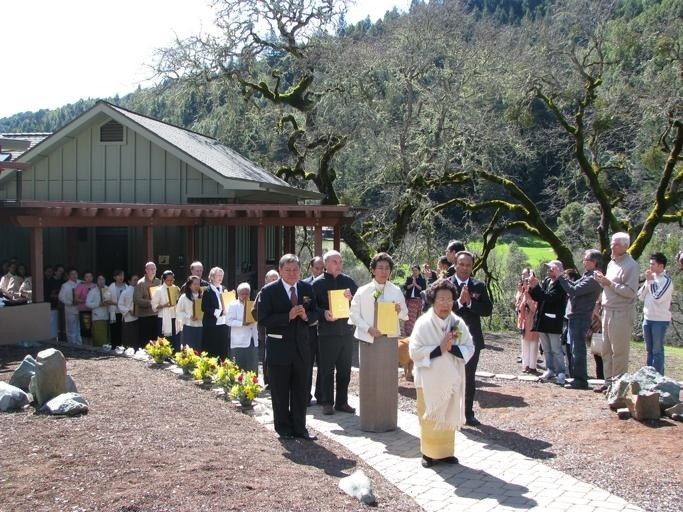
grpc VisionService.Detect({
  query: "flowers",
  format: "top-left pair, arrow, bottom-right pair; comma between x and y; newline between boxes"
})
175,344 -> 197,365
228,367 -> 265,398
216,354 -> 243,382
194,350 -> 218,374
146,334 -> 172,358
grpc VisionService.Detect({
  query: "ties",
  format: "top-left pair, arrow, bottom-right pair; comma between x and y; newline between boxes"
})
290,287 -> 298,306
460,283 -> 466,288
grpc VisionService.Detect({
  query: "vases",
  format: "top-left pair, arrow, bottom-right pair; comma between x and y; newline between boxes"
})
181,361 -> 197,379
200,371 -> 215,387
153,353 -> 168,366
239,392 -> 256,408
223,379 -> 236,396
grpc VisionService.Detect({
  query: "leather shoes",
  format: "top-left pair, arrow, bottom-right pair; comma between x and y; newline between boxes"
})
282,426 -> 318,440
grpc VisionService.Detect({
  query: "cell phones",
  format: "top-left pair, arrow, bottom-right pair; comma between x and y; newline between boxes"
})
533,271 -> 535,277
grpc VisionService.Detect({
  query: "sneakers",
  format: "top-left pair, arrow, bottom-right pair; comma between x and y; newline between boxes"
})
522,366 -> 588,390
323,404 -> 334,415
334,403 -> 355,413
466,418 -> 480,426
438,456 -> 458,464
422,455 -> 433,468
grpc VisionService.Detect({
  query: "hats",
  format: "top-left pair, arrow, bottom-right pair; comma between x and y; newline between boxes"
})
543,260 -> 563,271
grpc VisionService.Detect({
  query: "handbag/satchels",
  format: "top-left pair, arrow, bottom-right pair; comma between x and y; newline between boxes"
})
590,332 -> 603,357
